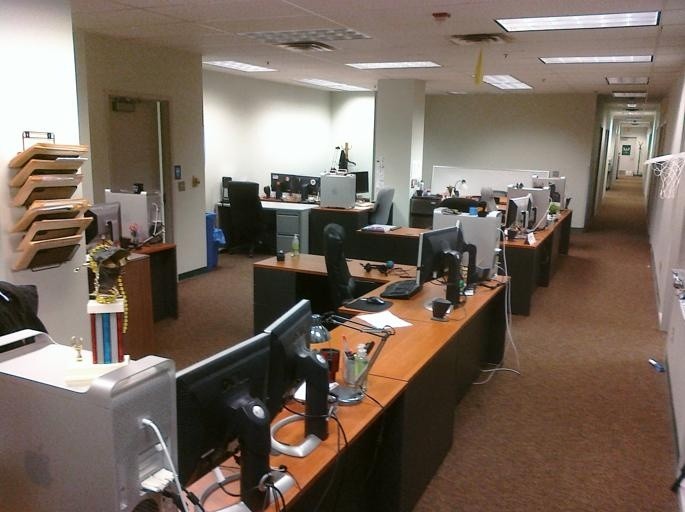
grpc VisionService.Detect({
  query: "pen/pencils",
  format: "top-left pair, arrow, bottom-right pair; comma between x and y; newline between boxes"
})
342,330 -> 389,386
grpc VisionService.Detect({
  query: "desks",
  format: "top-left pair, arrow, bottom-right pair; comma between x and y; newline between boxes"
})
115,239 -> 177,360
309,201 -> 394,256
357,195 -> 573,316
216,198 -> 320,253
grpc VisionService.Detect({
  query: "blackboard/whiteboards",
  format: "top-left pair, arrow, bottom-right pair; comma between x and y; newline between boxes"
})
431,166 -> 550,198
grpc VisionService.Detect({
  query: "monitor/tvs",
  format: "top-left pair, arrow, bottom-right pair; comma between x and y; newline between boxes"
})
84,202 -> 122,243
175,332 -> 295,512
263,298 -> 329,458
416,184 -> 560,314
270,171 -> 369,205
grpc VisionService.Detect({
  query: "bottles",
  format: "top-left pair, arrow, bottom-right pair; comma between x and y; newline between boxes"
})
419,179 -> 424,191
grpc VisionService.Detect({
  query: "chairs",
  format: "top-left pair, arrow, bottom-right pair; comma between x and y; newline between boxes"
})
227,181 -> 269,257
371,188 -> 395,224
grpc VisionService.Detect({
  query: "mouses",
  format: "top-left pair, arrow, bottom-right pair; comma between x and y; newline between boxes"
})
368,296 -> 384,305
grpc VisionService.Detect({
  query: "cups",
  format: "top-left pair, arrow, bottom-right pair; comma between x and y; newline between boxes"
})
417,190 -> 423,196
133,182 -> 144,193
469,207 -> 478,215
343,355 -> 367,387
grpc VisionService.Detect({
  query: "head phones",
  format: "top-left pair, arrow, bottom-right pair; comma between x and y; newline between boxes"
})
365,263 -> 385,274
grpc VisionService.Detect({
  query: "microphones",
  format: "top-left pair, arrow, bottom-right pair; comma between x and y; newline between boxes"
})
360,262 -> 364,267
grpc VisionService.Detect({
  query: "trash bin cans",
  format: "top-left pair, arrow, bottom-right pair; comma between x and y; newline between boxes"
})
206,212 -> 218,269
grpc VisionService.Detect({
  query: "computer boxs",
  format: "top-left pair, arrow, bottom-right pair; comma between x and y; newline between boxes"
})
0,329 -> 179,512
104,191 -> 163,244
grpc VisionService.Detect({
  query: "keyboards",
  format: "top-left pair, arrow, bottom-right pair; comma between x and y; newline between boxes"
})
380,280 -> 422,300
